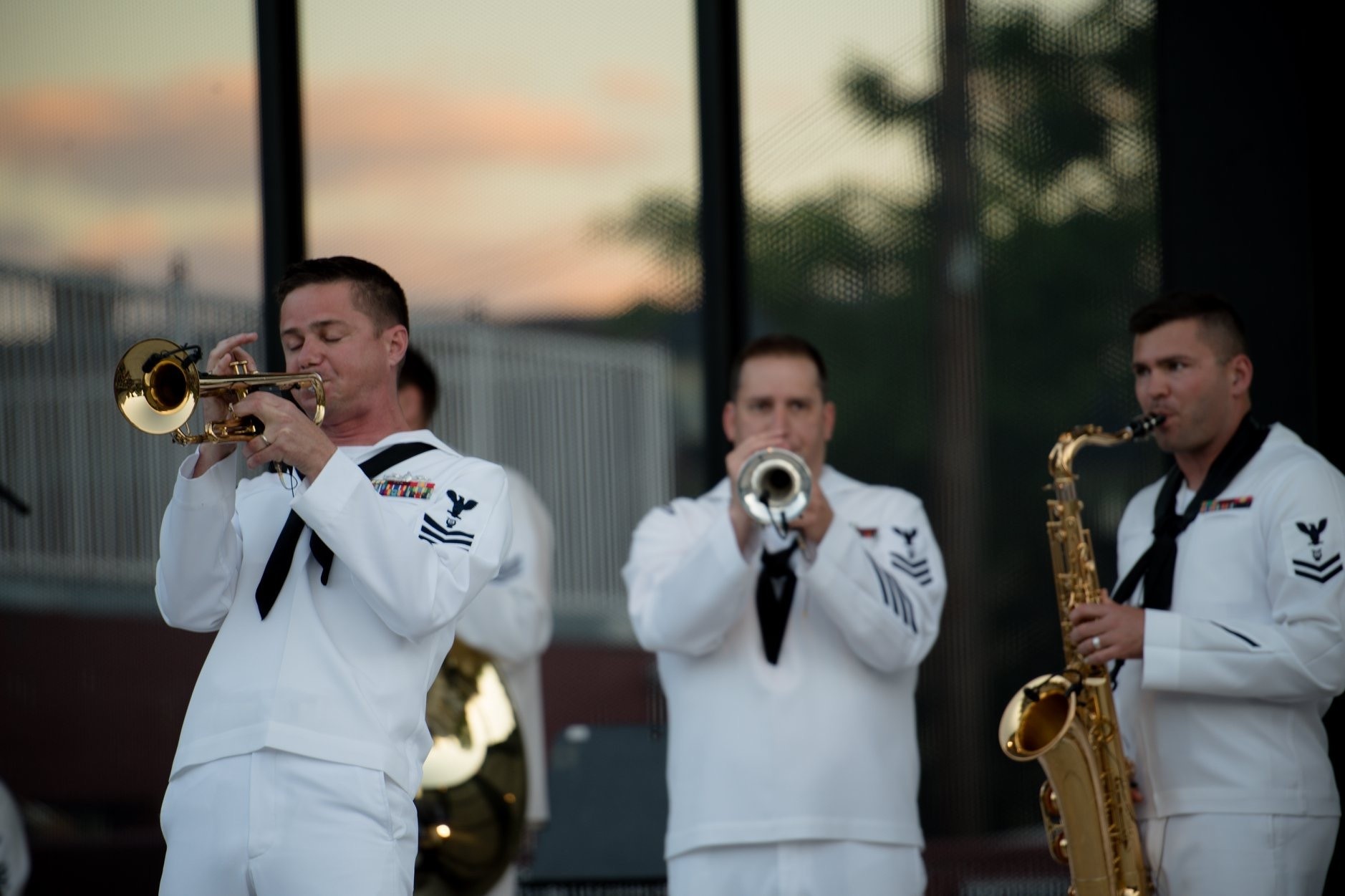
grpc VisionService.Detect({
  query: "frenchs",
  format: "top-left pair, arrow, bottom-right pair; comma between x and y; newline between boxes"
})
412,637 -> 531,894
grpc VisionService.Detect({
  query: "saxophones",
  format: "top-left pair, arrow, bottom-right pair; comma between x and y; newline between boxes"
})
998,408 -> 1169,895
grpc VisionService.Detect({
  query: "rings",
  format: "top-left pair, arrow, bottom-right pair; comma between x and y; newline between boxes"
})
260,434 -> 269,447
1093,636 -> 1102,652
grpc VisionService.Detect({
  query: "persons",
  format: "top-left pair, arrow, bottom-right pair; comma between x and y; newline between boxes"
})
1065,285 -> 1345,895
618,332 -> 949,893
152,256 -> 514,896
392,341 -> 554,895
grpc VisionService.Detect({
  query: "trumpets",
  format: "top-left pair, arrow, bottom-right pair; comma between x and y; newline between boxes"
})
738,445 -> 815,536
112,338 -> 327,448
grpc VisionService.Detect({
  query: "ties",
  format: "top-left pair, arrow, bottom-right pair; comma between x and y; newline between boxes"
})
255,442 -> 437,620
756,537 -> 798,666
1102,403 -> 1274,693
